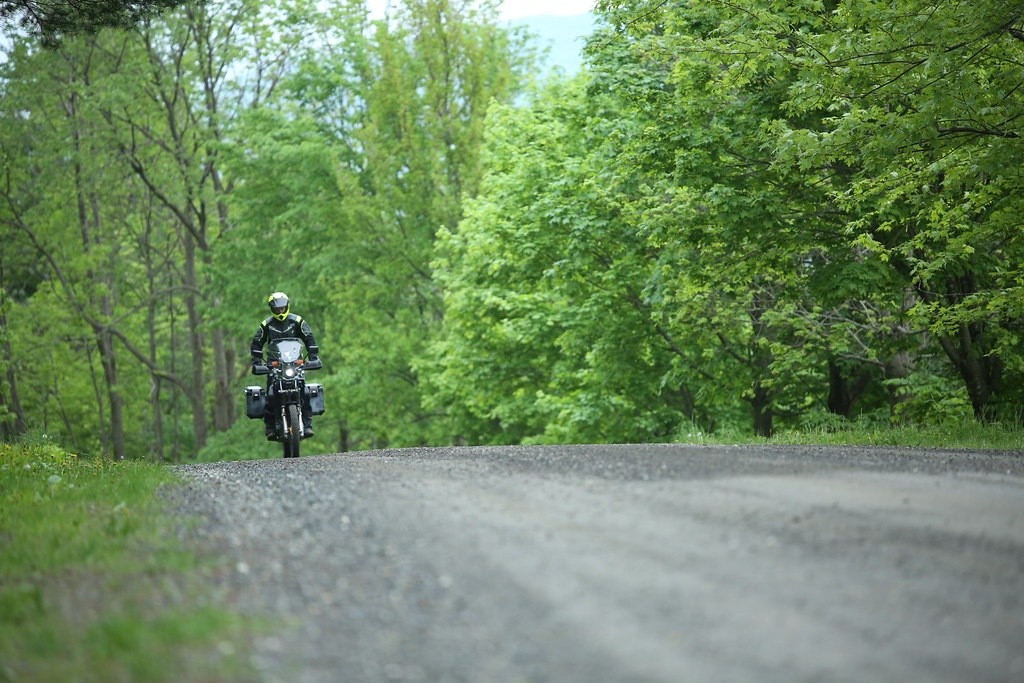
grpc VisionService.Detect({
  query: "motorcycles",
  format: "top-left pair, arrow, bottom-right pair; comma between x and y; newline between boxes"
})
244,345 -> 325,458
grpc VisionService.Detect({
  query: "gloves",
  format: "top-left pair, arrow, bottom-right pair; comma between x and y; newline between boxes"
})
310,355 -> 318,361
252,356 -> 262,366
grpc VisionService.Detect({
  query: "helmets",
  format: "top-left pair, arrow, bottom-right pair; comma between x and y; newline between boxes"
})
267,292 -> 289,321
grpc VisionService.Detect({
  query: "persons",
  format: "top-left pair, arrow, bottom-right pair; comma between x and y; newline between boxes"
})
251,292 -> 318,441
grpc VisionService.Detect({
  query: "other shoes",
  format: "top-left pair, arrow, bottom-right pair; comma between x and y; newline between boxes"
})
267,432 -> 277,441
304,428 -> 314,437
